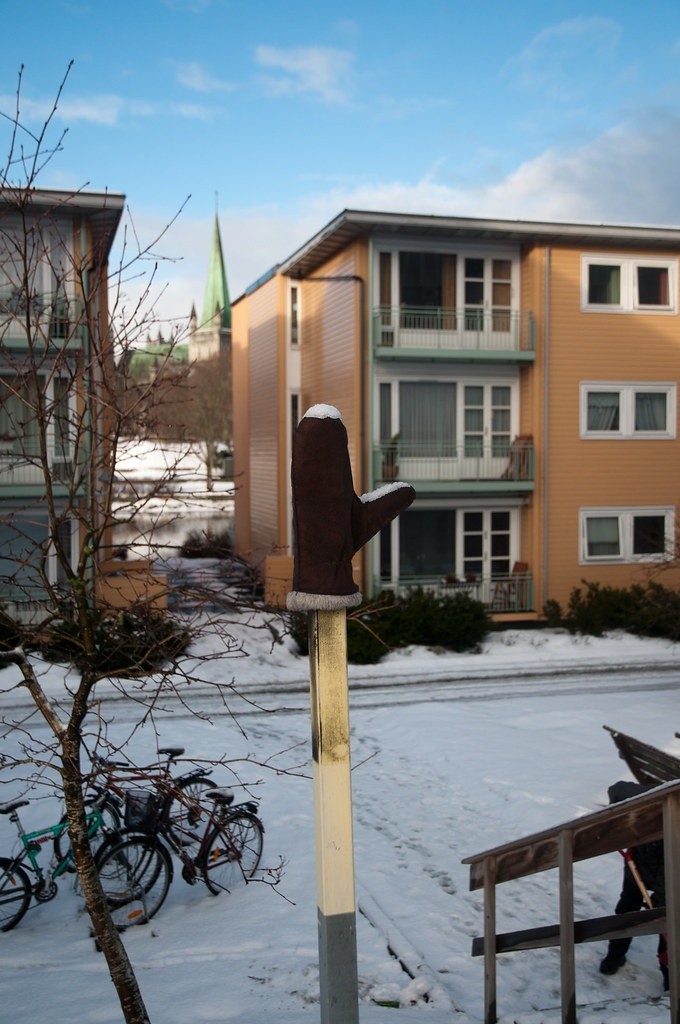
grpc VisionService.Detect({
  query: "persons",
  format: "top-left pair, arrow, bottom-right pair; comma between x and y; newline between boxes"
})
598,781 -> 671,992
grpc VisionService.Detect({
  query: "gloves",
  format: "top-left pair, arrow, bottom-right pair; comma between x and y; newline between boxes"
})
285,404 -> 417,611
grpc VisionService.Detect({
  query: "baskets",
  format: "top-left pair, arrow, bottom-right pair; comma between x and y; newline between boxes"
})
124,789 -> 155,830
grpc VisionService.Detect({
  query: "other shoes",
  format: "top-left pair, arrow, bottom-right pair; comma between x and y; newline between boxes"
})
660,966 -> 670,991
599,954 -> 626,974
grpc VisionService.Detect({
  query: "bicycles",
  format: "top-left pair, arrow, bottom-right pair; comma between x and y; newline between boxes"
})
0,744 -> 265,933
